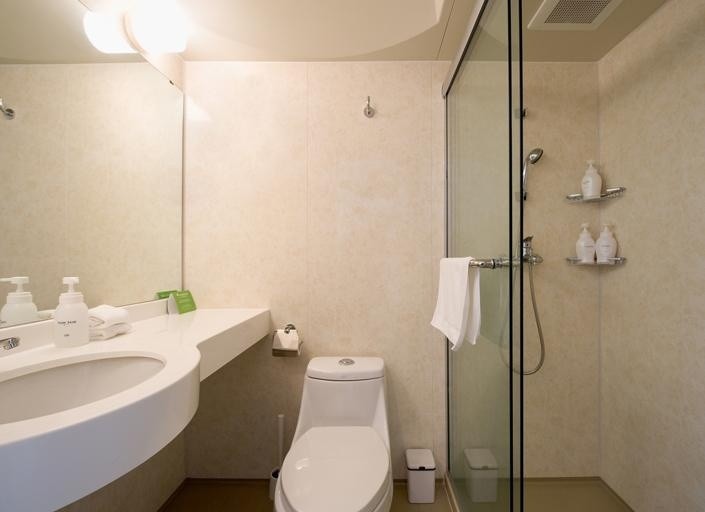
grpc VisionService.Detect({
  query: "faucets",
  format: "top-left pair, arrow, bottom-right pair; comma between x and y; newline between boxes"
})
3,336 -> 20,351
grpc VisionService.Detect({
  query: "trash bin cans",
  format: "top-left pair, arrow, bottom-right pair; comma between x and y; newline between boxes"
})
405,449 -> 436,504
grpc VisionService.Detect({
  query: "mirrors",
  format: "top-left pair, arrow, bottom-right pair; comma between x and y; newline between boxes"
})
0,0 -> 183,329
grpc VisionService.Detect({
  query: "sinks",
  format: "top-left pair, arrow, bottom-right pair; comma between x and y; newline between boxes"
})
0,349 -> 167,427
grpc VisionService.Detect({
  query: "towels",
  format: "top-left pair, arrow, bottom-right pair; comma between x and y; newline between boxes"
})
87,303 -> 131,341
431,257 -> 483,353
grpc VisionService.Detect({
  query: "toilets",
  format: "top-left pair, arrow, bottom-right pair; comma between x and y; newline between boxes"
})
274,358 -> 394,512
